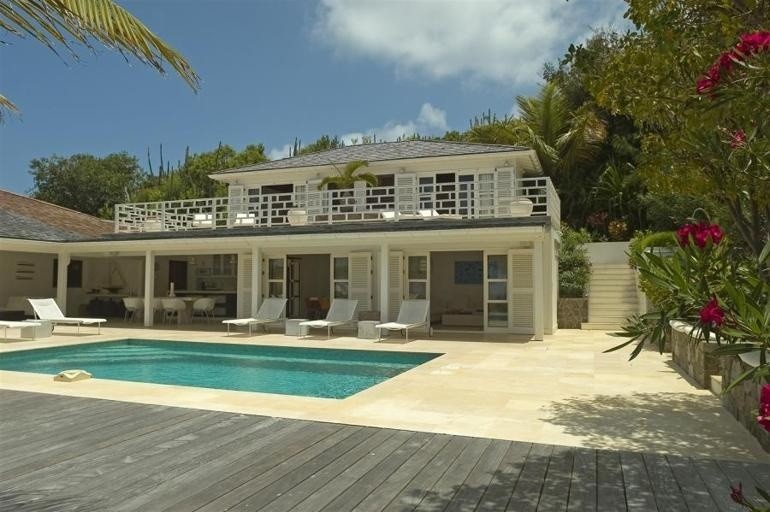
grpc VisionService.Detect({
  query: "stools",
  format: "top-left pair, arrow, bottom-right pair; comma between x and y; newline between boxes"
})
143,218 -> 165,232
286,208 -> 308,225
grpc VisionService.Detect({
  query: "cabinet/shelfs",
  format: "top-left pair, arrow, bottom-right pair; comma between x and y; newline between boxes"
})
191,252 -> 238,280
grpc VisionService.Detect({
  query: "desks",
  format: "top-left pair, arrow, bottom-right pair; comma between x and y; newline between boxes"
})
284,318 -> 311,337
357,319 -> 384,340
20,319 -> 53,339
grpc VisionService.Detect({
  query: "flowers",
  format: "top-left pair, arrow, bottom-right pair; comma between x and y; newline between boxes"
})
600,27 -> 770,441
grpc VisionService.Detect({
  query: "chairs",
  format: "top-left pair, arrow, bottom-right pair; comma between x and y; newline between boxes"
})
378,207 -> 422,221
0,319 -> 40,343
299,296 -> 360,341
377,297 -> 435,344
26,297 -> 107,337
419,206 -> 463,220
232,210 -> 257,228
121,286 -> 219,331
190,213 -> 214,229
222,296 -> 289,337
510,198 -> 534,217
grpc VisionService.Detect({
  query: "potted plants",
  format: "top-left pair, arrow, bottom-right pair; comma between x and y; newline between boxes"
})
556,244 -> 588,331
561,224 -> 592,323
315,156 -> 381,212
